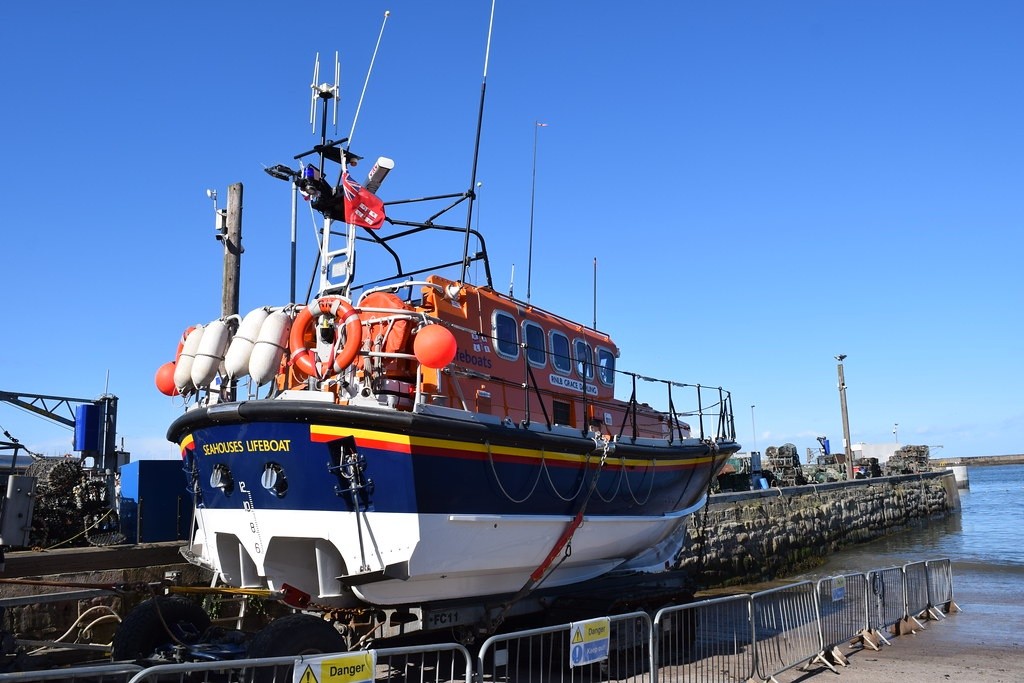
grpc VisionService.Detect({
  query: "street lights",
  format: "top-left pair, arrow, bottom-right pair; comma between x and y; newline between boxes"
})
834,353 -> 854,481
750,404 -> 755,451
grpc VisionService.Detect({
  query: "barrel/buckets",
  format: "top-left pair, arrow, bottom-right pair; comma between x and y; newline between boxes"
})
759,478 -> 769,489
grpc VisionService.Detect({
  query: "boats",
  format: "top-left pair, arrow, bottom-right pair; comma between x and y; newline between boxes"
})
155,0 -> 743,654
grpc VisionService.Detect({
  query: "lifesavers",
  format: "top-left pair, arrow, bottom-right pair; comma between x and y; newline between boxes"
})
246,612 -> 351,683
287,298 -> 362,378
112,596 -> 211,663
176,325 -> 196,369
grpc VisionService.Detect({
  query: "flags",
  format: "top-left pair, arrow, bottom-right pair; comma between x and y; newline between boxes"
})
340,168 -> 385,230
